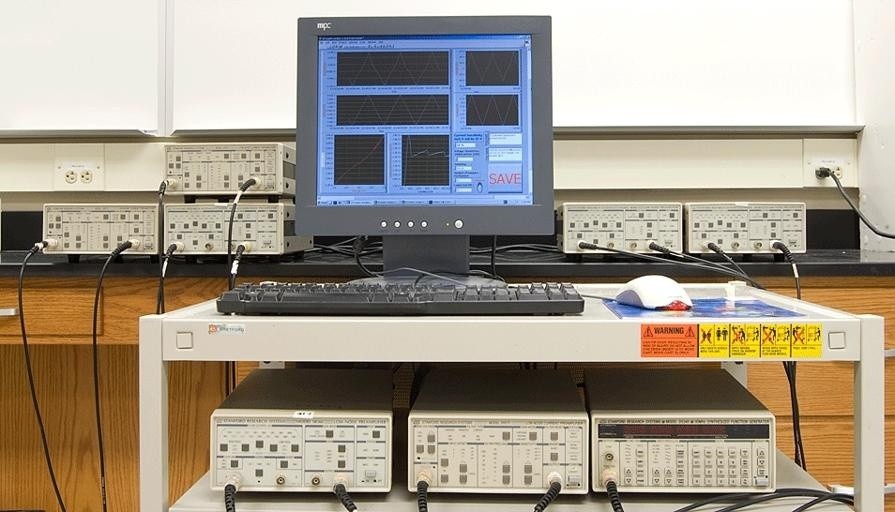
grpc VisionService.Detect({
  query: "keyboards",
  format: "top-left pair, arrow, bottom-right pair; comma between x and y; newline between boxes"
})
216,280 -> 586,316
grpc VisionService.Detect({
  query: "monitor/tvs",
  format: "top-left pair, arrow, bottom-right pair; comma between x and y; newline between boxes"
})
294,13 -> 556,285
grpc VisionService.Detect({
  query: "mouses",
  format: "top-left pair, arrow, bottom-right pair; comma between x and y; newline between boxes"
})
615,273 -> 693,311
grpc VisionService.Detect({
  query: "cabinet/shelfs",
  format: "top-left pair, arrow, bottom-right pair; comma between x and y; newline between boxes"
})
1,248 -> 895,512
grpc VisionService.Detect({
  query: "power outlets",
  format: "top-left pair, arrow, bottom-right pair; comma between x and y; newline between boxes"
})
803,139 -> 857,189
54,158 -> 104,191
